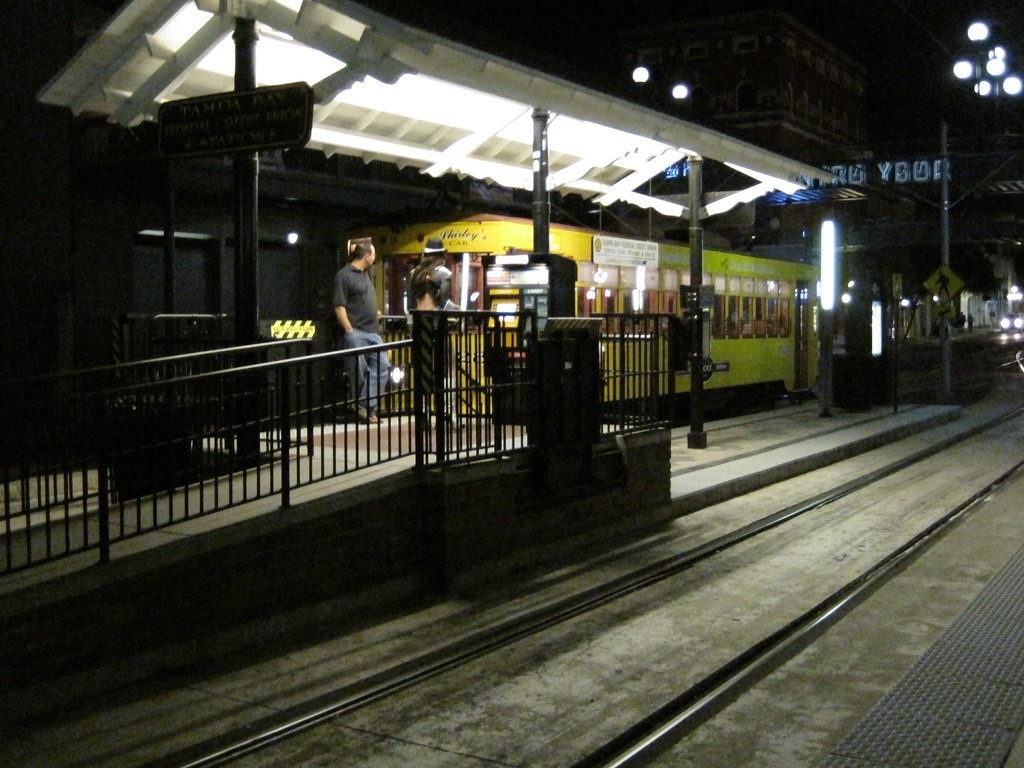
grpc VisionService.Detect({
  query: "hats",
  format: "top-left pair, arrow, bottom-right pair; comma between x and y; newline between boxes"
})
423,238 -> 448,254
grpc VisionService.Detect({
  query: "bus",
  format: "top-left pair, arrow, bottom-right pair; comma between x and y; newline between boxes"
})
333,212 -> 901,422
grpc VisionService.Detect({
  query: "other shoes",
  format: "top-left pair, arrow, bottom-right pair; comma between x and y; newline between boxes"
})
350,403 -> 369,420
366,411 -> 383,424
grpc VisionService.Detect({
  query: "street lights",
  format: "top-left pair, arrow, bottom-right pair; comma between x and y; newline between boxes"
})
926,22 -> 1022,396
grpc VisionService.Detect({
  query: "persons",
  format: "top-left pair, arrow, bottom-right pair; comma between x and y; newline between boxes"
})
408,238 -> 465,433
957,311 -> 975,333
598,317 -> 789,338
332,242 -> 389,424
